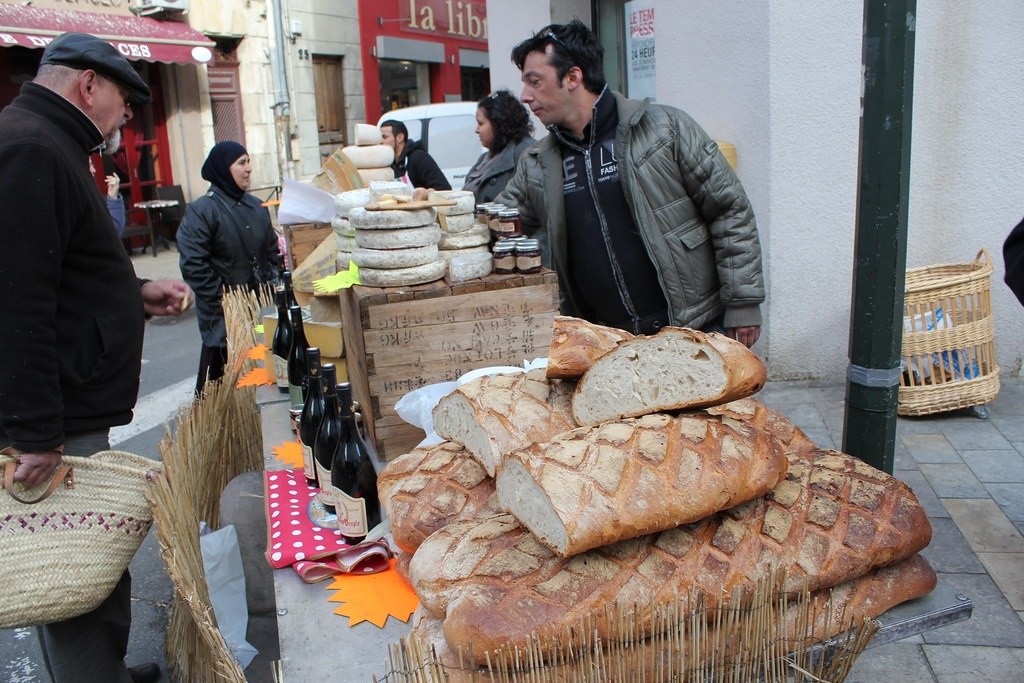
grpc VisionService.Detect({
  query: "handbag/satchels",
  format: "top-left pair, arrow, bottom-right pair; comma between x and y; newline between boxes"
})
0,451 -> 163,627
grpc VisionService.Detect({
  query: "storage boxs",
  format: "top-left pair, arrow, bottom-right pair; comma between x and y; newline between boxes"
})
283,223 -> 559,463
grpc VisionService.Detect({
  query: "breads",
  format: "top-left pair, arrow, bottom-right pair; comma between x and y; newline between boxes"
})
377,316 -> 937,683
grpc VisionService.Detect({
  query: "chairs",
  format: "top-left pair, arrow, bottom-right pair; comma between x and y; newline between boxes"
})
122,185 -> 191,257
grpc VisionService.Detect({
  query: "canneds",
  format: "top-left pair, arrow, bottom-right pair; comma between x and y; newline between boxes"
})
477,202 -> 542,275
288,400 -> 364,444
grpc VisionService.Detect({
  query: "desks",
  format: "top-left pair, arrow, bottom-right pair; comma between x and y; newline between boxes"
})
255,306 -> 973,683
134,200 -> 178,256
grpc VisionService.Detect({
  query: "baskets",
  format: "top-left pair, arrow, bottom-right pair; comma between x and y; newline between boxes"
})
897,249 -> 1001,416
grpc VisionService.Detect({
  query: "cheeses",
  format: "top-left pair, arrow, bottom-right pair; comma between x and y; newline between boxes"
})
334,123 -> 492,287
264,295 -> 349,383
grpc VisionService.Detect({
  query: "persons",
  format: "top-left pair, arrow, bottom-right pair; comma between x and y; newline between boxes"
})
462,89 -> 538,218
494,19 -> 765,348
381,120 -> 452,190
88,156 -> 124,238
0,31 -> 195,682
176,142 -> 283,390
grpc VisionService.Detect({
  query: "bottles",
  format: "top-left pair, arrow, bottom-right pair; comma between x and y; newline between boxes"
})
287,306 -> 310,407
314,363 -> 341,514
300,348 -> 324,488
331,382 -> 382,546
283,272 -> 299,310
272,290 -> 293,394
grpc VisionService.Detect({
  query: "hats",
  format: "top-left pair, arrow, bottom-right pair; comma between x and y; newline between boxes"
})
40,31 -> 153,104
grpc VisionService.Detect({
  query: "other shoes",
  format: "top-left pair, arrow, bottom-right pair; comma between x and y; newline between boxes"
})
127,663 -> 161,683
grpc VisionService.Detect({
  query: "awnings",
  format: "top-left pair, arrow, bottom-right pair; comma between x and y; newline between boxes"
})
0,3 -> 216,65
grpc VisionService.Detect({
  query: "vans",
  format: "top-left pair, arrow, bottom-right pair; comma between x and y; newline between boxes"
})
378,100 -> 489,189
